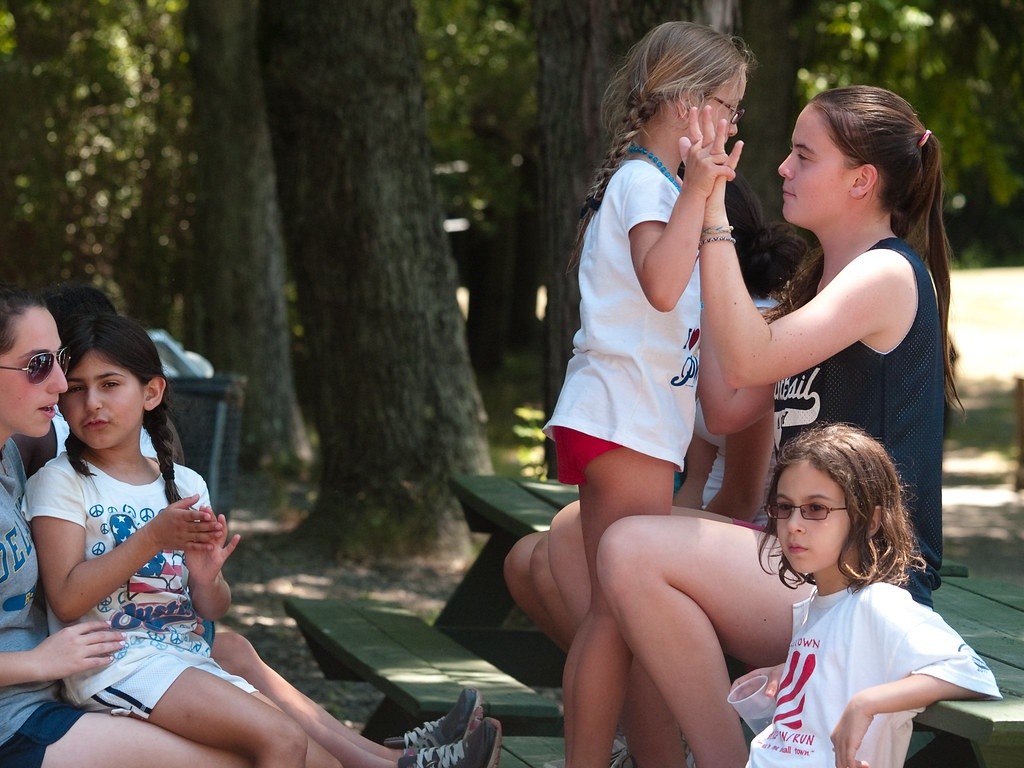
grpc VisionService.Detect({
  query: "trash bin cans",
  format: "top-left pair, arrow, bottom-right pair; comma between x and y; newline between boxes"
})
164,374 -> 247,525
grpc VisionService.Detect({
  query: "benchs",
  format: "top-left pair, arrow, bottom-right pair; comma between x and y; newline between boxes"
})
499,736 -> 565,768
285,599 -> 564,719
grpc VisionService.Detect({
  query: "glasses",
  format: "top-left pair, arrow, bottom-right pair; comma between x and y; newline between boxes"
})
703,91 -> 746,124
764,503 -> 849,520
0,347 -> 71,385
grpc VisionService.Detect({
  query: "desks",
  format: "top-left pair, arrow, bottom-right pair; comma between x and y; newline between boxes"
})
360,477 -> 1024,768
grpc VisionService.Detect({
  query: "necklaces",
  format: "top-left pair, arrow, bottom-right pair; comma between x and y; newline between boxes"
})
629,144 -> 708,310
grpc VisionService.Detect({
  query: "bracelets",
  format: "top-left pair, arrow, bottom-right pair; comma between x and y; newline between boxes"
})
700,225 -> 735,234
696,234 -> 739,245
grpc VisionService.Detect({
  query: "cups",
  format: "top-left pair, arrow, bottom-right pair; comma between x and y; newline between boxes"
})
727,676 -> 777,735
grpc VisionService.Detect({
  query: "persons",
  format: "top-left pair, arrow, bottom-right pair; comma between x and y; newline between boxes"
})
0,284 -> 503,768
502,169 -> 808,768
544,19 -> 754,768
22,309 -> 343,768
728,421 -> 1004,768
549,80 -> 966,768
11,286 -> 187,477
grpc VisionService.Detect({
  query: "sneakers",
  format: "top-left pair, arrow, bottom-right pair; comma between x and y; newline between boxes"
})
383,686 -> 484,756
398,717 -> 503,768
